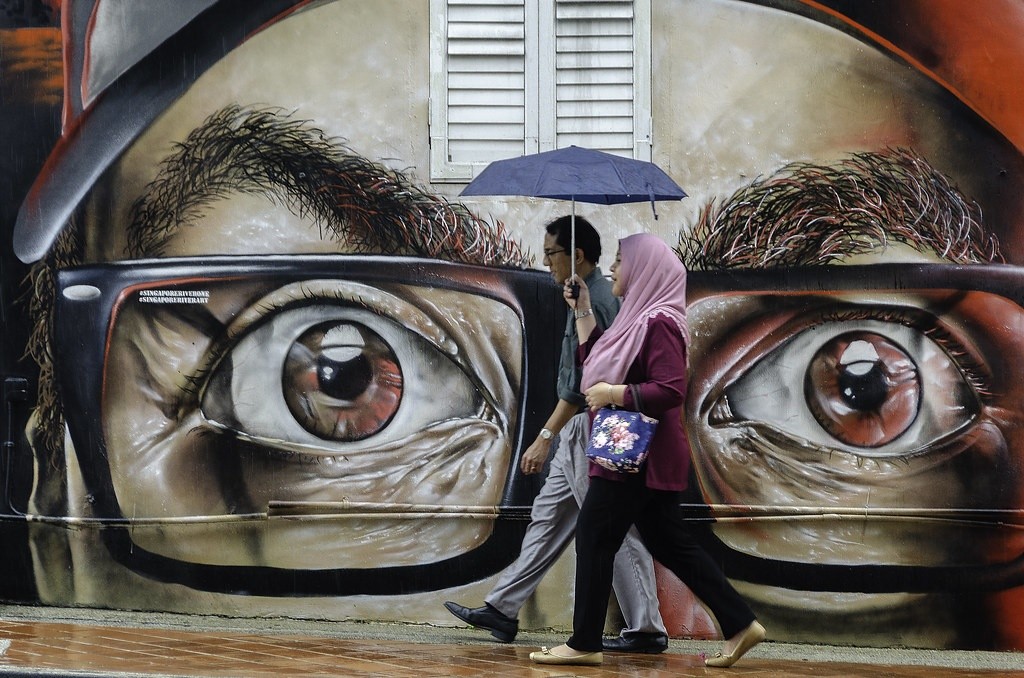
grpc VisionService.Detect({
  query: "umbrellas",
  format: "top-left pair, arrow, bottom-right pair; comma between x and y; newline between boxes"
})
458,144 -> 691,302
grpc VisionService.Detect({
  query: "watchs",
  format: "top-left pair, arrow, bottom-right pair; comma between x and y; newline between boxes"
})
540,429 -> 555,440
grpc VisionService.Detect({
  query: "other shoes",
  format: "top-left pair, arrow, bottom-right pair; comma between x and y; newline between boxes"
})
530,645 -> 604,665
704,620 -> 767,669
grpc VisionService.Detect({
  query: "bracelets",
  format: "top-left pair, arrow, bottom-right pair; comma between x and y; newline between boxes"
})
608,384 -> 616,410
575,309 -> 593,319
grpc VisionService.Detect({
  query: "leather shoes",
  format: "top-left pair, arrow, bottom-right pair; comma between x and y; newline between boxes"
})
601,632 -> 668,655
442,601 -> 519,643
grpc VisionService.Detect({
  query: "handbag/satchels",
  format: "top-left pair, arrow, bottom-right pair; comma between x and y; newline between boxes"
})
585,383 -> 659,474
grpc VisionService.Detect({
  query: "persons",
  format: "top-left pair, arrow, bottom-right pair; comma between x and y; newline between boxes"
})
442,215 -> 669,654
529,231 -> 767,667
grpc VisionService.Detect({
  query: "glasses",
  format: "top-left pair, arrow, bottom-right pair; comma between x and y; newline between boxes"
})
543,249 -> 569,260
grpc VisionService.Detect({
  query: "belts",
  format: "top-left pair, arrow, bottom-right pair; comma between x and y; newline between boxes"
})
574,404 -> 589,415
